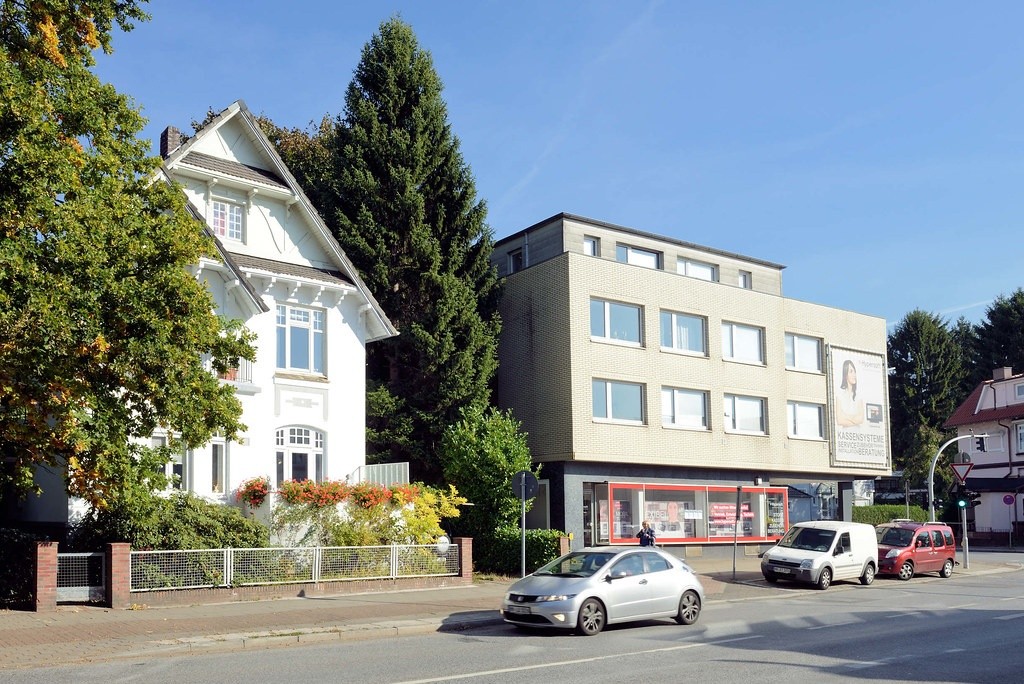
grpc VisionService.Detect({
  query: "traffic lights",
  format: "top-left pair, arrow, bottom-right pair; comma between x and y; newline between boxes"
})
976,432 -> 988,452
966,489 -> 982,509
956,486 -> 968,509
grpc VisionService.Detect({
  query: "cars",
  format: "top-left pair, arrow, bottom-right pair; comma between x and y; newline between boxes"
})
499,544 -> 705,636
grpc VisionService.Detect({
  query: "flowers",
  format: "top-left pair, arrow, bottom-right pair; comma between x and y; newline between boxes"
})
236,477 -> 272,510
276,479 -> 417,508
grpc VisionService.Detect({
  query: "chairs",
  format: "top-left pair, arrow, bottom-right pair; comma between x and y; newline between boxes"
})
595,556 -> 606,566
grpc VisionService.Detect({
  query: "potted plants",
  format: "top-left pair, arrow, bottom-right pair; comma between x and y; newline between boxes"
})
218,366 -> 237,381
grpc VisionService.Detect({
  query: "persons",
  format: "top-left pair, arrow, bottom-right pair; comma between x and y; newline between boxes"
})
657,500 -> 681,530
613,562 -> 635,578
834,360 -> 866,428
636,521 -> 656,547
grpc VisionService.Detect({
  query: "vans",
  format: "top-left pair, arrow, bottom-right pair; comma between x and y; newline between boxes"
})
873,518 -> 957,581
760,520 -> 880,591
